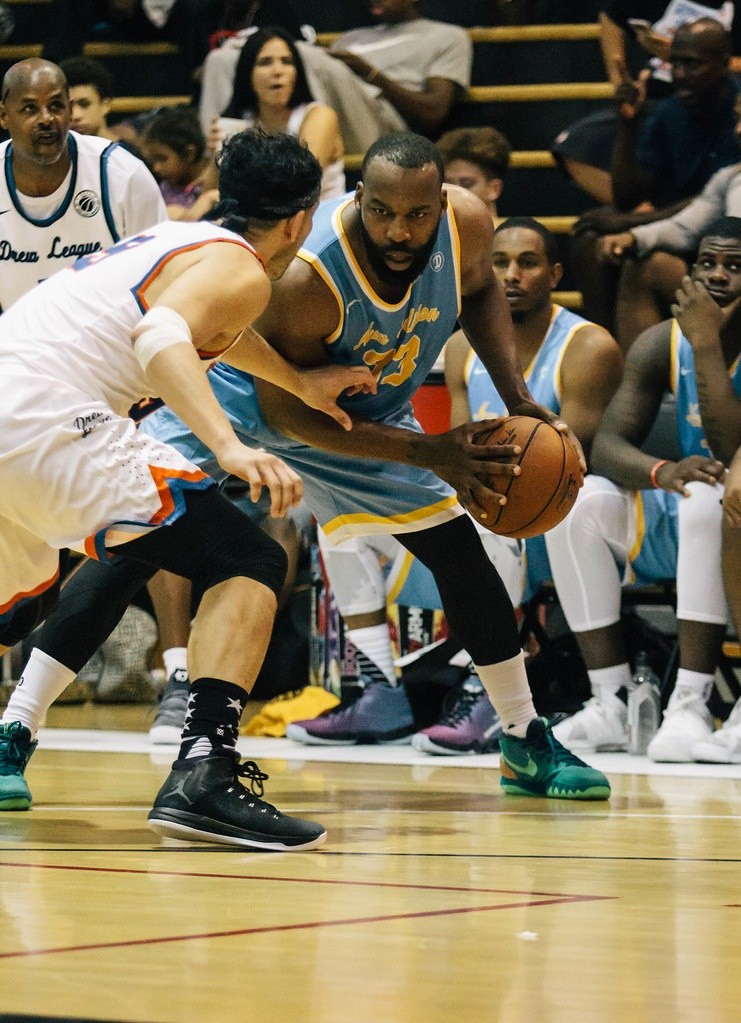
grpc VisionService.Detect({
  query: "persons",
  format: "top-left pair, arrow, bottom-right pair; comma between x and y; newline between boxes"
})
0,125 -> 380,851
0,0 -> 741,762
0,131 -> 611,811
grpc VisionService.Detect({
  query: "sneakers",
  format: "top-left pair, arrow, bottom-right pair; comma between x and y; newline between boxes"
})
647,668 -> 714,762
0,720 -> 38,811
551,662 -> 633,753
285,674 -> 417,744
692,694 -> 741,766
412,679 -> 502,755
148,668 -> 190,744
147,748 -> 327,851
499,716 -> 611,798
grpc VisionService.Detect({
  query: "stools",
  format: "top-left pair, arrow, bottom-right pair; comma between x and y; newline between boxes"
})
509,582 -> 741,721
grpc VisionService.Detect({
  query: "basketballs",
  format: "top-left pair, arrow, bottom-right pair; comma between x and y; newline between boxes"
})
462,414 -> 582,540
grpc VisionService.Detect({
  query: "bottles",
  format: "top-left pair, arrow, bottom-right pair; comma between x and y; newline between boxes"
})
627,652 -> 661,755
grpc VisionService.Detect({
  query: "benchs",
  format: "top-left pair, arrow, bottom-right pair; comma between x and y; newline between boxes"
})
0,16 -> 626,314
